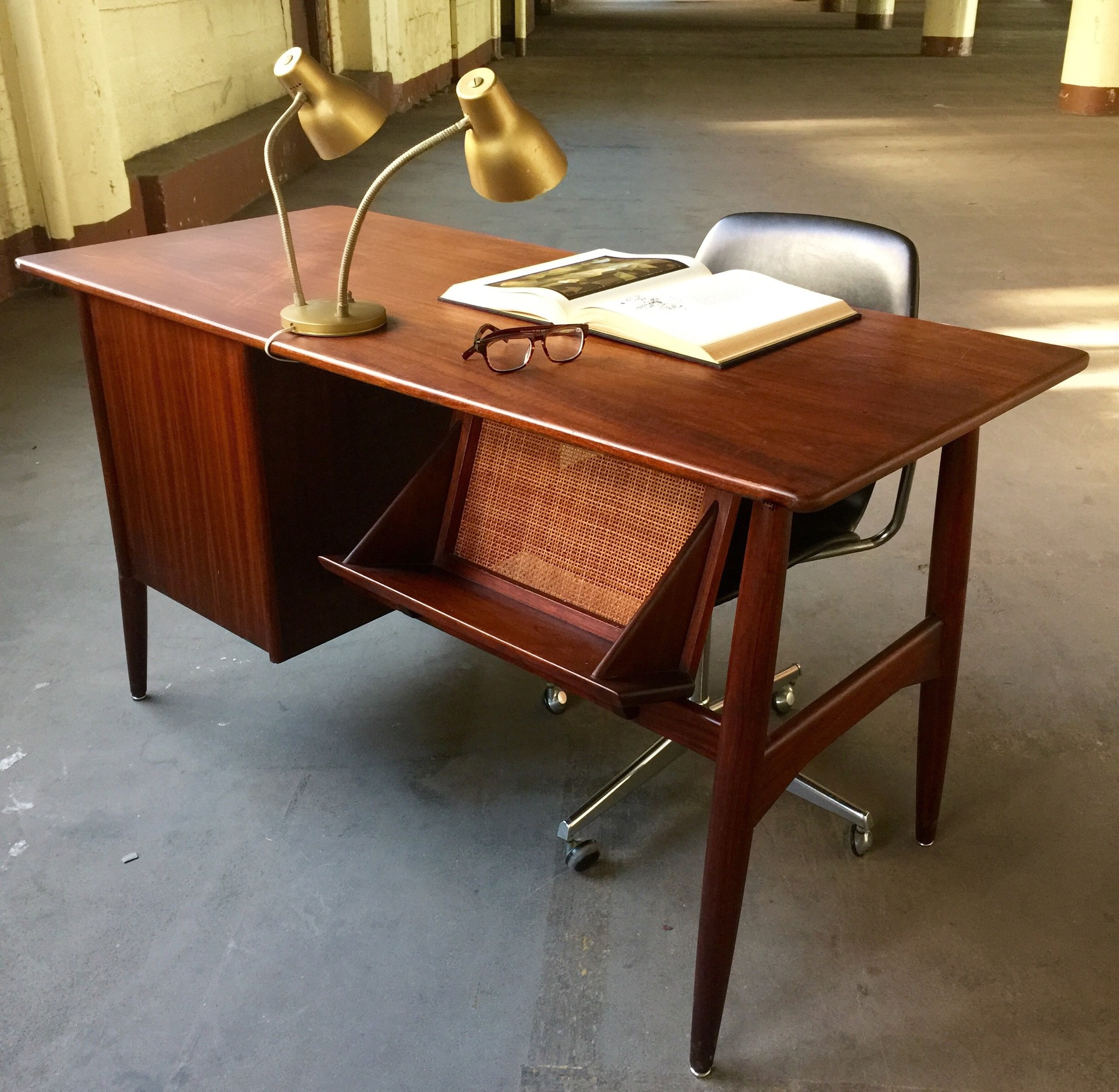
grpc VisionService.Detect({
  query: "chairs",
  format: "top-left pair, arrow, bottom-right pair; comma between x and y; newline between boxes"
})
542,211 -> 921,872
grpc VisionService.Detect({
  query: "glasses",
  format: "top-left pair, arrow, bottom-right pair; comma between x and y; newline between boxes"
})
463,322 -> 591,373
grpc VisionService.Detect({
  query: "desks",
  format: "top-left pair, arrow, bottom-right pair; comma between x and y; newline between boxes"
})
14,205 -> 1090,1078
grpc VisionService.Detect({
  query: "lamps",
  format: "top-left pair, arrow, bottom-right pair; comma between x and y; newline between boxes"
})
264,46 -> 568,337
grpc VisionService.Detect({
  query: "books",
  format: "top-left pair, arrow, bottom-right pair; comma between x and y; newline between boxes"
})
437,243 -> 866,369
